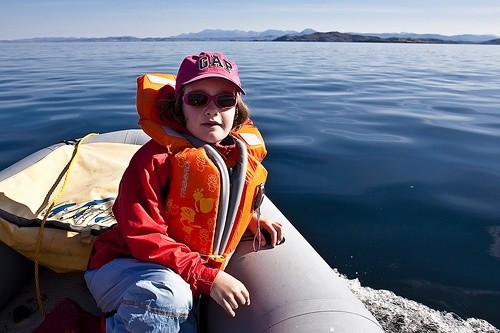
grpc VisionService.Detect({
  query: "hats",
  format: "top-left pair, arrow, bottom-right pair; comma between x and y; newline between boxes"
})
172,51 -> 246,99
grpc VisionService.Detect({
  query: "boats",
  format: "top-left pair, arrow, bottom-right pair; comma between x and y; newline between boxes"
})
0,129 -> 386,333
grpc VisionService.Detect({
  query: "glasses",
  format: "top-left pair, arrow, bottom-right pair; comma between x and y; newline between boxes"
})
179,90 -> 240,110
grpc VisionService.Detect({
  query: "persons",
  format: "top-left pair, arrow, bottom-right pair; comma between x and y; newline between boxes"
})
82,49 -> 286,333
